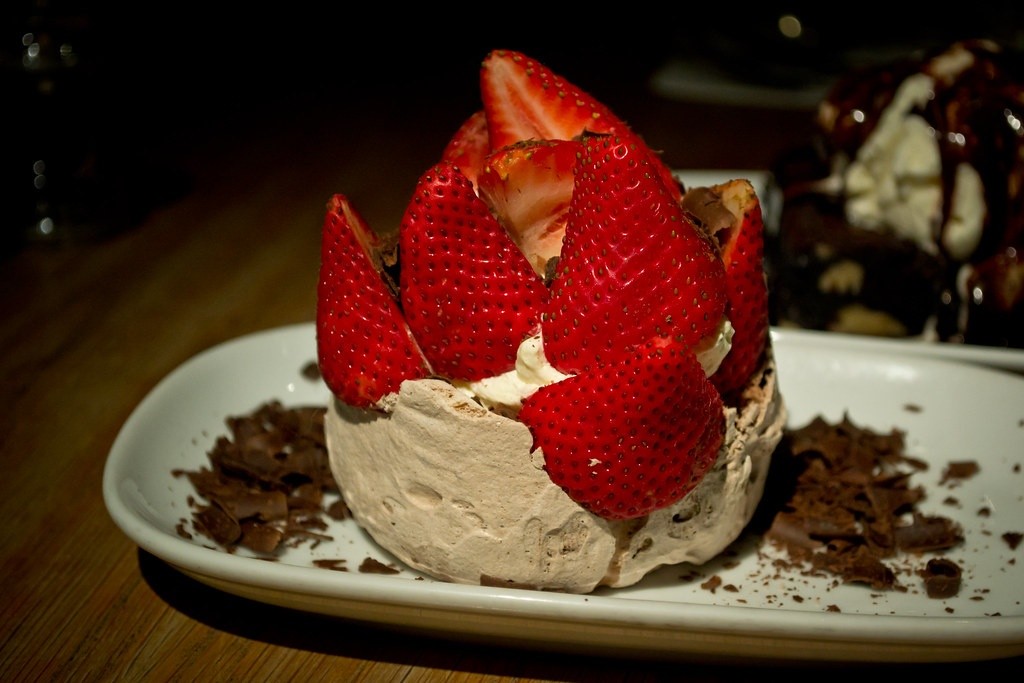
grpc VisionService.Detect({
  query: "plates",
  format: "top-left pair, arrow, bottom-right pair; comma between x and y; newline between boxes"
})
99,309 -> 1023,667
585,158 -> 1024,376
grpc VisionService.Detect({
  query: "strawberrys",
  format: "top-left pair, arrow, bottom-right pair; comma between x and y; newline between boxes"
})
309,43 -> 774,523
